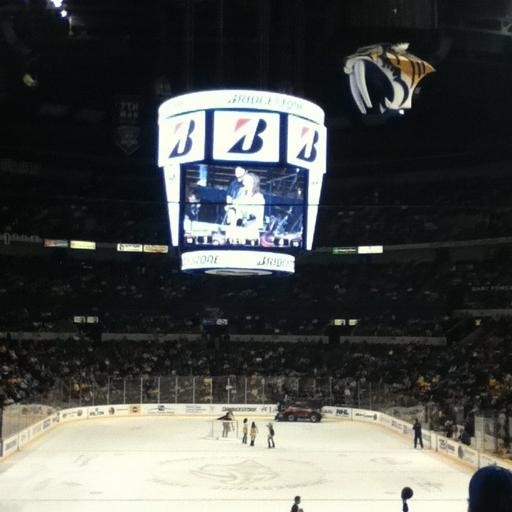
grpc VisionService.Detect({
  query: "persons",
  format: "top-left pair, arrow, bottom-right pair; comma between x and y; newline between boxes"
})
182,187 -> 217,230
227,172 -> 268,242
290,495 -> 303,512
463,464 -> 511,511
219,164 -> 249,210
1,244 -> 512,457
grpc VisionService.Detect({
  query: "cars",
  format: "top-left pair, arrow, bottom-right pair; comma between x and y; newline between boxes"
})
282,402 -> 322,422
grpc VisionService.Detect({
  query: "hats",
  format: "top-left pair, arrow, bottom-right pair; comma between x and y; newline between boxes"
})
469,466 -> 512,508
